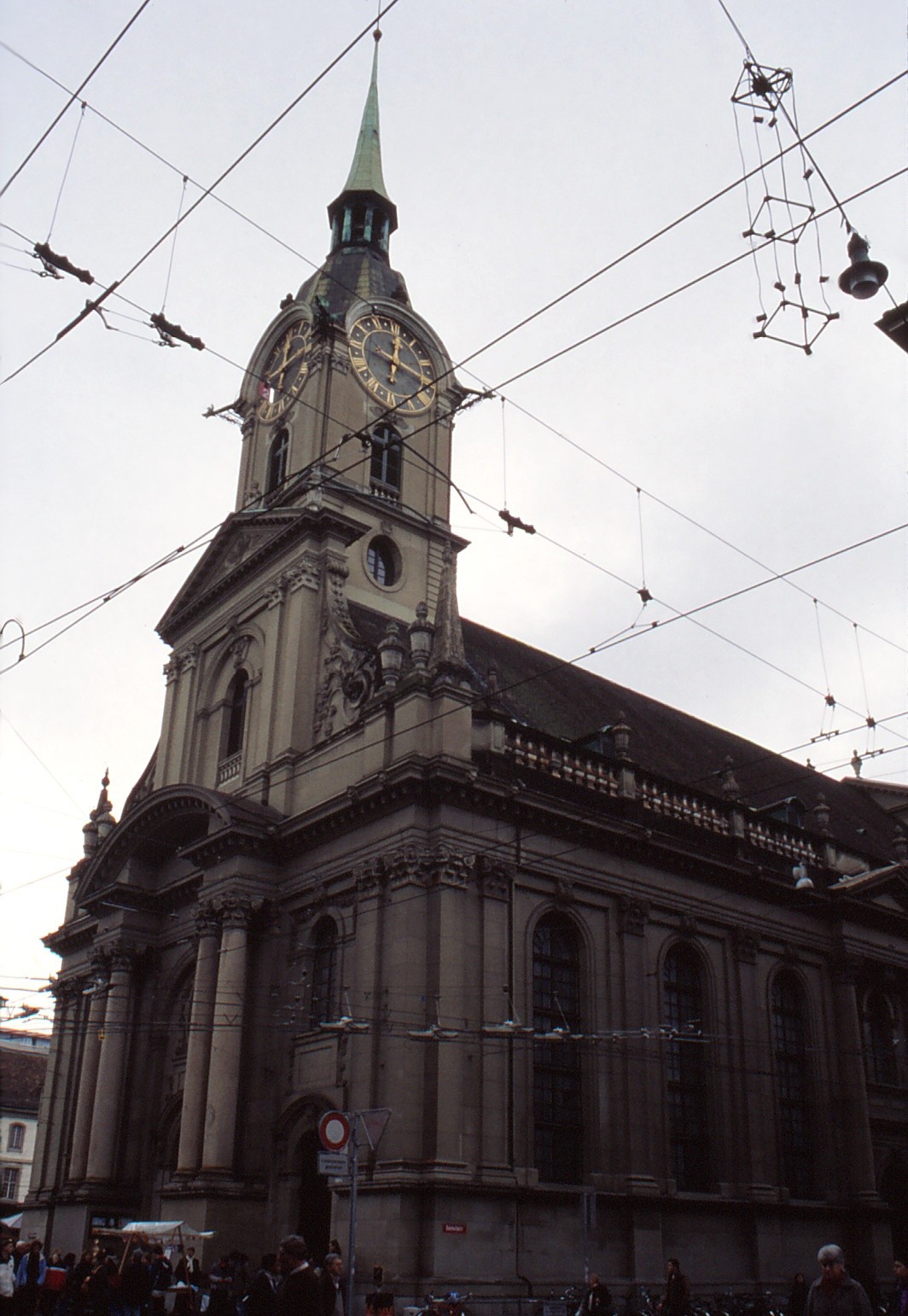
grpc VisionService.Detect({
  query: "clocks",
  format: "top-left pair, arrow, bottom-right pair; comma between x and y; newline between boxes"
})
347,315 -> 438,416
255,319 -> 311,423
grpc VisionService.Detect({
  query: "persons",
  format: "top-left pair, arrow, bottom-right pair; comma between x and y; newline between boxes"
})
277,1237 -> 321,1316
889,1254 -> 908,1316
308,1239 -> 344,1316
248,1254 -> 280,1316
805,1244 -> 872,1316
175,1247 -> 201,1312
660,1258 -> 687,1316
45,1247 -> 174,1316
0,1237 -> 47,1316
585,1274 -> 613,1316
788,1272 -> 810,1316
206,1251 -> 249,1316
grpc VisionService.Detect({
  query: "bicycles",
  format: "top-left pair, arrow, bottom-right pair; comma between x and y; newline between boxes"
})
412,1274 -> 786,1316
